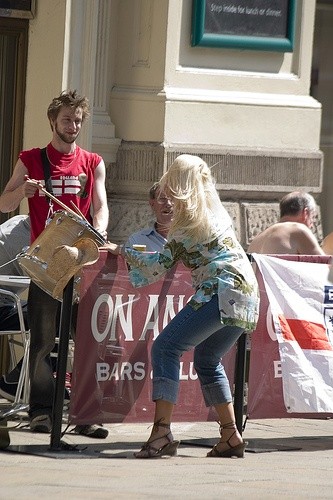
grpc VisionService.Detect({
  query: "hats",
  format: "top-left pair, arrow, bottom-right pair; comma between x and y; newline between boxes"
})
47,238 -> 98,299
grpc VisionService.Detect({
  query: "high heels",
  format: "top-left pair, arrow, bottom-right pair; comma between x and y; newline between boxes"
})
134,417 -> 179,459
206,420 -> 248,458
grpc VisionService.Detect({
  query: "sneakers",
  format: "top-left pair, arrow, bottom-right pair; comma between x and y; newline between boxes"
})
0,382 -> 25,404
75,424 -> 108,438
30,414 -> 52,433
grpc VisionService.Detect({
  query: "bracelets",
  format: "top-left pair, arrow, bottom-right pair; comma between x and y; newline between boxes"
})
118,243 -> 124,255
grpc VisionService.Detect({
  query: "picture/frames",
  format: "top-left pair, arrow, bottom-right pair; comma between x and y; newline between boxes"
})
192,0 -> 297,52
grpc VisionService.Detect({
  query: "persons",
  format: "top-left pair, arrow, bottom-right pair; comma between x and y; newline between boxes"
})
124,180 -> 177,256
98,153 -> 260,459
0,91 -> 110,439
246,190 -> 329,256
0,214 -> 73,405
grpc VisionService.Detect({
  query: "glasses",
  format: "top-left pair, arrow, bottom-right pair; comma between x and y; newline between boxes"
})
154,197 -> 172,205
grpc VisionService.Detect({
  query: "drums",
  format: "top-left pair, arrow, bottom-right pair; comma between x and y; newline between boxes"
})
18,209 -> 109,306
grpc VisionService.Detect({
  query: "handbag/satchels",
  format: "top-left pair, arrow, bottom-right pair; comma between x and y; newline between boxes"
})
0,413 -> 22,448
